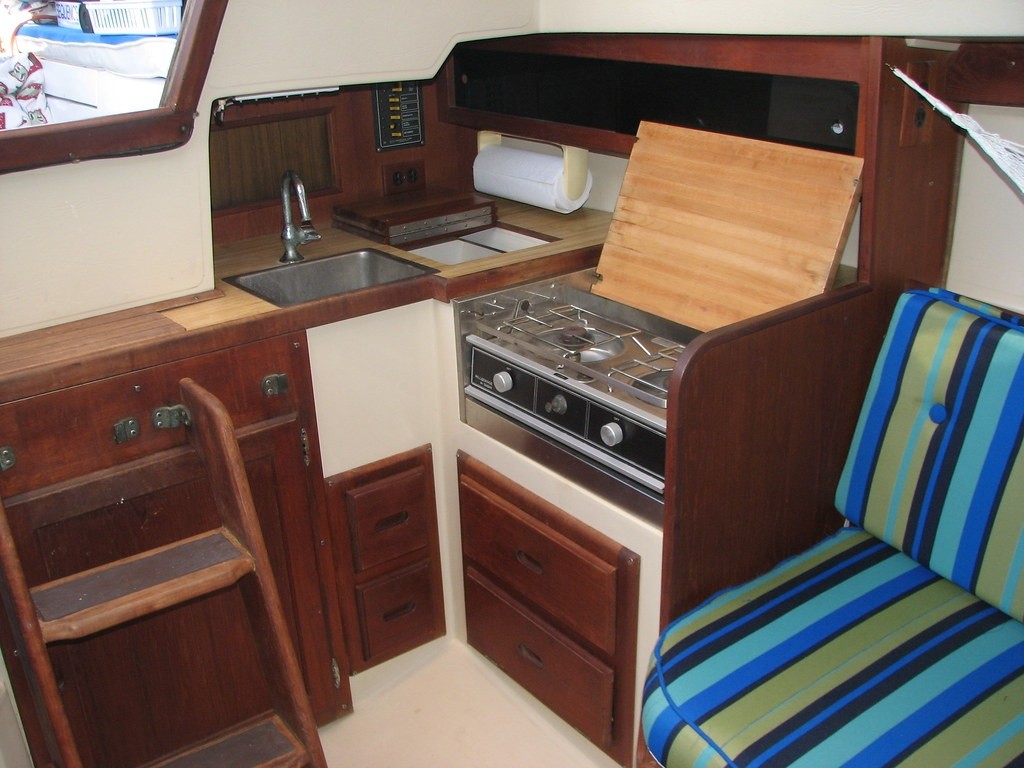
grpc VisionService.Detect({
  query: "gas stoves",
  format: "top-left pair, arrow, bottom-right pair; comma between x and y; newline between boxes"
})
450,267 -> 706,532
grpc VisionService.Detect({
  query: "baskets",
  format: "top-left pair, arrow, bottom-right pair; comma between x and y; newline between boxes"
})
82,1 -> 184,36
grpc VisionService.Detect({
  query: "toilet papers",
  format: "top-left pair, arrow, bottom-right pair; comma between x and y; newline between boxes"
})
472,145 -> 594,215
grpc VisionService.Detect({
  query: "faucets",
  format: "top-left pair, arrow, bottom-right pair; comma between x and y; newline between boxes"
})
277,171 -> 322,264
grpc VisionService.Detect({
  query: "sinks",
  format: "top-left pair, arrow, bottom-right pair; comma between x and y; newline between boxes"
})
220,243 -> 441,308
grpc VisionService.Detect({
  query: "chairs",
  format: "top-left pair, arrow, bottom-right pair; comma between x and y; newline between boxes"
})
630,287 -> 1024,768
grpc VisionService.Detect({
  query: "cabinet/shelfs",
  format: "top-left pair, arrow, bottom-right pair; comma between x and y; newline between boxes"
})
455,453 -> 641,768
320,448 -> 446,676
0,331 -> 353,768
